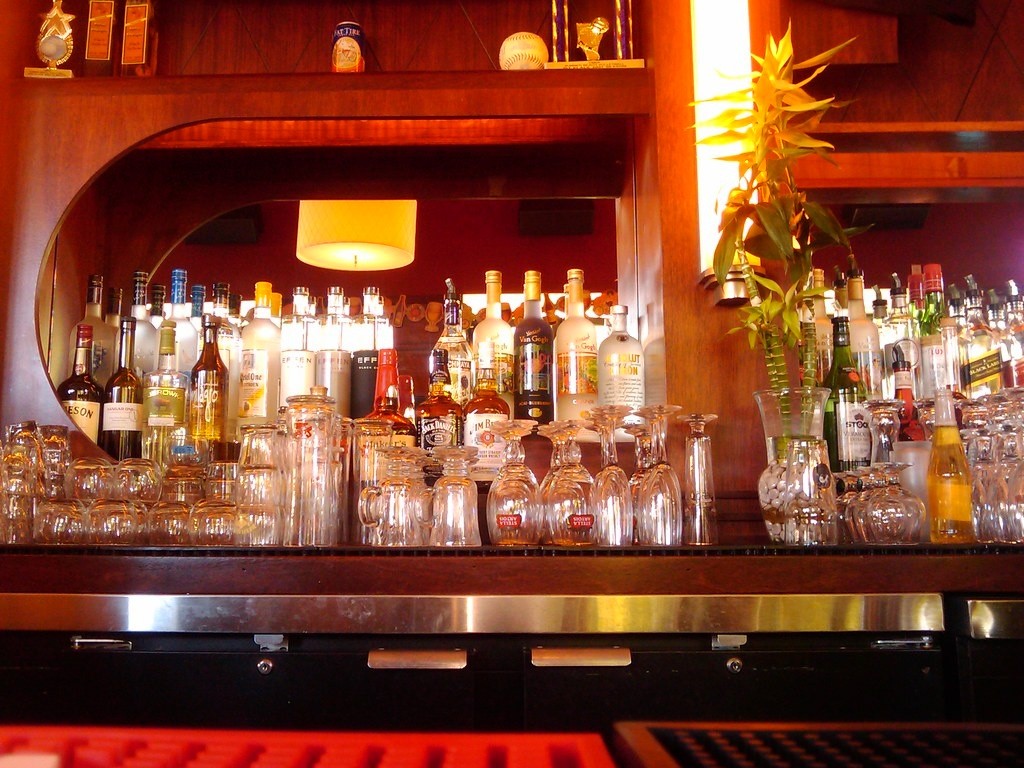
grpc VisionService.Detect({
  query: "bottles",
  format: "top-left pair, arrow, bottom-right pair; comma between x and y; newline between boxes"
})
59,267 -> 655,486
929,388 -> 981,544
791,257 -> 1023,417
887,359 -> 924,441
814,315 -> 874,488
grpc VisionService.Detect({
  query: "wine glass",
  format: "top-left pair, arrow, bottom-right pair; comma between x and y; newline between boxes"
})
359,406 -> 721,542
830,460 -> 926,544
966,384 -> 1024,543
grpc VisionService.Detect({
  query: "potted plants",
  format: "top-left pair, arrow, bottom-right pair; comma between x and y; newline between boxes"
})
685,11 -> 874,545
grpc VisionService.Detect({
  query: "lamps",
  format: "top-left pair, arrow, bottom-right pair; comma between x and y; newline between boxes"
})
295,200 -> 417,271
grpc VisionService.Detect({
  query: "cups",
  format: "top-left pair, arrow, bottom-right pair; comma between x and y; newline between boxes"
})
0,386 -> 354,547
783,438 -> 839,545
